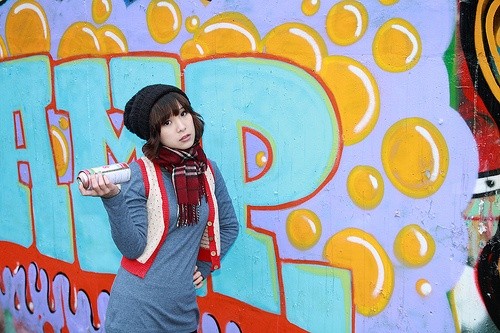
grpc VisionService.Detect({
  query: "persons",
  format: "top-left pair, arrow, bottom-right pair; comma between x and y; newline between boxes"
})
70,83 -> 241,333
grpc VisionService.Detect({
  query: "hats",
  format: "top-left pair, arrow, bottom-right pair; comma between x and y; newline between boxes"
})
123,83 -> 192,141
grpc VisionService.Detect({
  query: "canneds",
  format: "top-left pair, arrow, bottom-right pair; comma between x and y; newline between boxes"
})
77,162 -> 131,191
